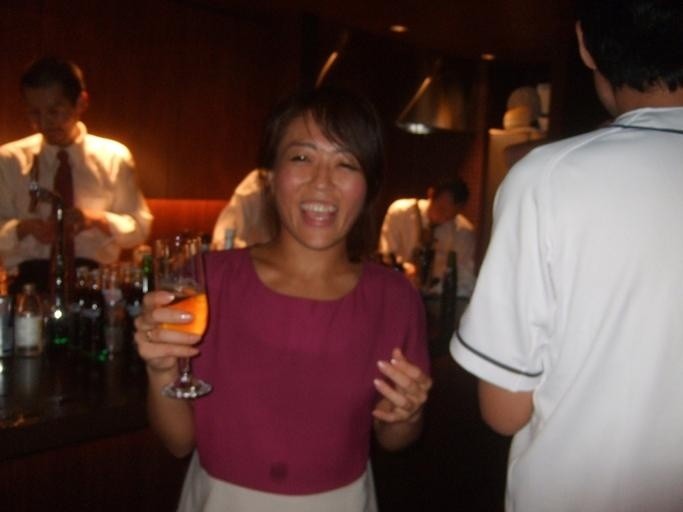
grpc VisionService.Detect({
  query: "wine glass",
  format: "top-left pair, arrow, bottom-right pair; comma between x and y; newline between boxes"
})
152,236 -> 217,401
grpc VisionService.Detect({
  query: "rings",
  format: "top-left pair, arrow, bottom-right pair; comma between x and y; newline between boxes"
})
144,329 -> 153,343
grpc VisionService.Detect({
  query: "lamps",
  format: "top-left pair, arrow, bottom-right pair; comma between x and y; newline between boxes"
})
391,53 -> 468,136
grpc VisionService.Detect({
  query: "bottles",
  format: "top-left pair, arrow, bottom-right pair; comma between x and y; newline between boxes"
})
0,253 -> 157,362
441,245 -> 458,324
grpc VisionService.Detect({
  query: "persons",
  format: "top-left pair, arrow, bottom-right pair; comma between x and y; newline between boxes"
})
442,1 -> 680,511
204,161 -> 285,255
127,87 -> 439,512
0,55 -> 158,303
371,173 -> 479,297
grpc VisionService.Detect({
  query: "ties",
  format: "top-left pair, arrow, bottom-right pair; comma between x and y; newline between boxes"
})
51,150 -> 74,227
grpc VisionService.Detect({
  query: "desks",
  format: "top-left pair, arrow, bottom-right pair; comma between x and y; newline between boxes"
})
1,346 -> 150,460
